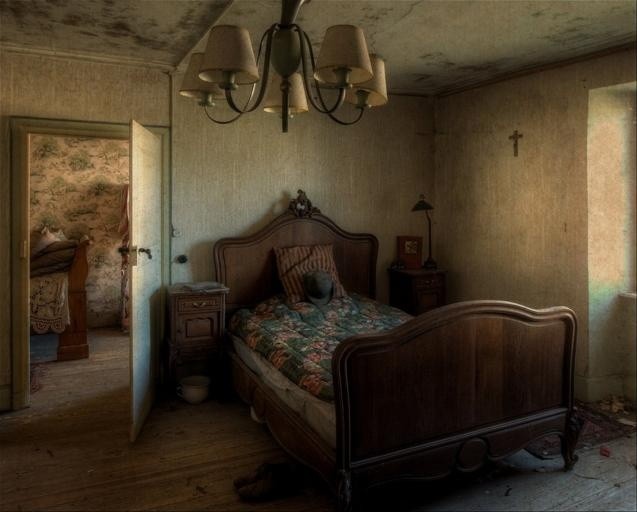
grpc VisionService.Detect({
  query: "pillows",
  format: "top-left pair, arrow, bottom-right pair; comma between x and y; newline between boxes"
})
31,228 -> 75,273
272,241 -> 349,304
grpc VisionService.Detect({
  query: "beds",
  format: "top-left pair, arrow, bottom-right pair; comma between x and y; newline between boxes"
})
27,226 -> 94,359
212,192 -> 579,506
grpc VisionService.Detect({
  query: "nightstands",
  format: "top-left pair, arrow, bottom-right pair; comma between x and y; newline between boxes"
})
166,283 -> 230,378
387,267 -> 448,316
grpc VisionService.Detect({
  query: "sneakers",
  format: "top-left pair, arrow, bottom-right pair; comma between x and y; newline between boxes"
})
233,462 -> 305,502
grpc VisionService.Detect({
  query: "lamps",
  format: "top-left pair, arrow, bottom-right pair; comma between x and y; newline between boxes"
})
175,0 -> 387,130
408,195 -> 438,269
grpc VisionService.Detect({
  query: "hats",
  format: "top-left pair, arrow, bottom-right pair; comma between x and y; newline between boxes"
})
303,270 -> 334,305
